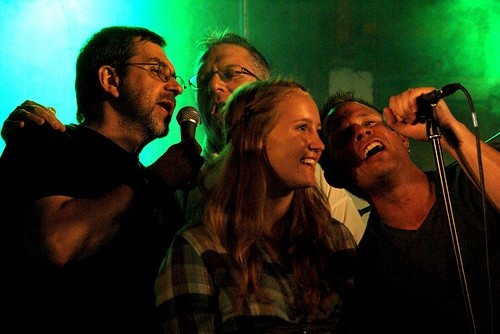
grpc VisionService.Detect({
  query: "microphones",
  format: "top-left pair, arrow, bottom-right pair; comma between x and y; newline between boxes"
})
176,106 -> 200,143
383,83 -> 459,128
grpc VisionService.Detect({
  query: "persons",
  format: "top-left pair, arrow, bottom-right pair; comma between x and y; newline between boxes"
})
317,86 -> 500,334
0,26 -> 365,334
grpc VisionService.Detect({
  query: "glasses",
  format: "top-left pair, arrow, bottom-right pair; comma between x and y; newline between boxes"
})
189,65 -> 263,91
122,62 -> 187,90
